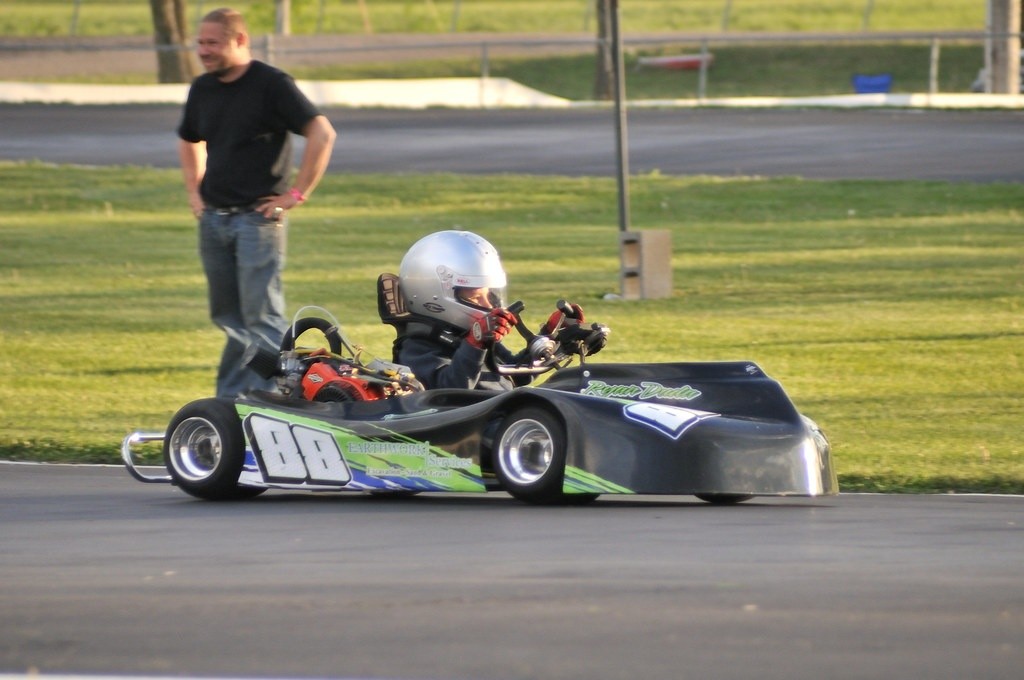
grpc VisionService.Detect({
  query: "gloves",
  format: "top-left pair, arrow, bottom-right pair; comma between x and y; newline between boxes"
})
542,304 -> 584,336
467,308 -> 518,349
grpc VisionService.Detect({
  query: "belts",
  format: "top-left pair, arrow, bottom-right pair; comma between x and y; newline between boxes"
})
208,204 -> 257,216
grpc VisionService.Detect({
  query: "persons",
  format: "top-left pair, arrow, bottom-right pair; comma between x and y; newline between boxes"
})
399,231 -> 584,407
178,9 -> 337,399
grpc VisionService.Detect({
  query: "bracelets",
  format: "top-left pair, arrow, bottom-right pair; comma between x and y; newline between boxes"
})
288,186 -> 308,204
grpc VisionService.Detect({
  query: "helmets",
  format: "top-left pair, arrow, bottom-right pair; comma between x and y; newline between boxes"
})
399,229 -> 506,330
